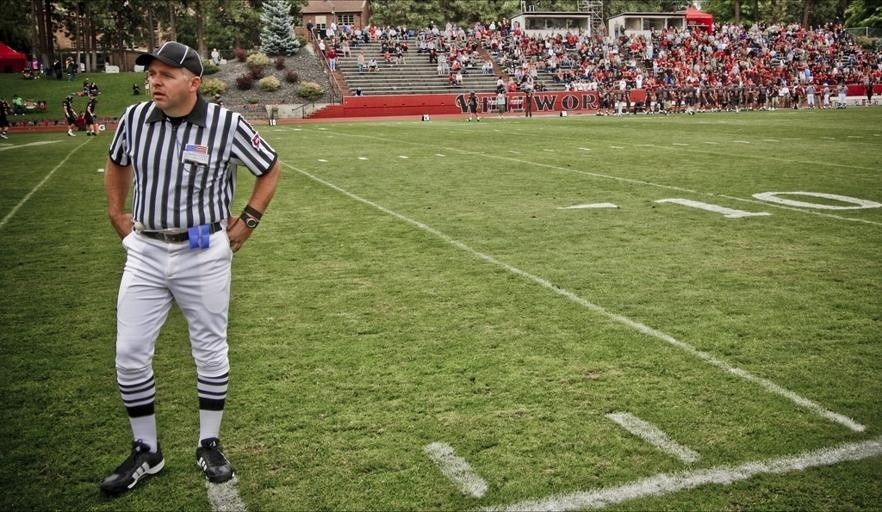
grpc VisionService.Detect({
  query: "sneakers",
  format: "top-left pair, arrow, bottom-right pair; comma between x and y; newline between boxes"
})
87,132 -> 100,136
196,437 -> 232,483
67,130 -> 76,136
100,440 -> 165,493
1,132 -> 9,139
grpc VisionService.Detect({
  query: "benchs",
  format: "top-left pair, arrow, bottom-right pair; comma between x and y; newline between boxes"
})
329,39 -> 566,94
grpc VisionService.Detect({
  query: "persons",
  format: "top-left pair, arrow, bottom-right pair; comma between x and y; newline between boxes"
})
468,91 -> 480,122
21,56 -> 79,81
0,91 -> 46,117
355,87 -> 362,96
213,90 -> 223,105
0,97 -> 10,141
318,22 -> 409,72
62,77 -> 102,137
132,82 -> 142,95
143,71 -> 151,96
416,8 -> 882,117
98,42 -> 281,499
211,48 -> 221,66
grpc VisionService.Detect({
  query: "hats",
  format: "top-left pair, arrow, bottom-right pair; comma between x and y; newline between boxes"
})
135,41 -> 203,78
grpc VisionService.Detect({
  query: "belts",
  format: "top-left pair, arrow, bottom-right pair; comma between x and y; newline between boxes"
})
134,221 -> 222,242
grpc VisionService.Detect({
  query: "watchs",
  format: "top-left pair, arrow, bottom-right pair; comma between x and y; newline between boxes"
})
240,212 -> 259,230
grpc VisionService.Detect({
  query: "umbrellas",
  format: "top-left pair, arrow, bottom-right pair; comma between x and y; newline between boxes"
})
1,43 -> 27,76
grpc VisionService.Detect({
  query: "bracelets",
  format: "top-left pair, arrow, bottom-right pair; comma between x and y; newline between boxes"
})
244,204 -> 262,221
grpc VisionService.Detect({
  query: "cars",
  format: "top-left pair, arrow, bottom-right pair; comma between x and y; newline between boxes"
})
40,58 -> 86,73
